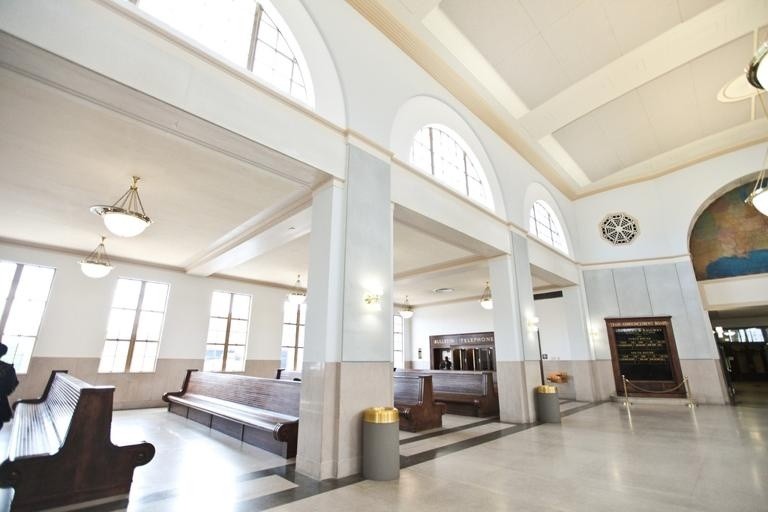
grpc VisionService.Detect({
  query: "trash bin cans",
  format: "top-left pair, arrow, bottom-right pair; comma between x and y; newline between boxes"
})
362,406 -> 400,481
536,384 -> 561,423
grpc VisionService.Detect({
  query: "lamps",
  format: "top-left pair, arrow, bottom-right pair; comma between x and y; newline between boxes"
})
743,143 -> 768,217
744,41 -> 768,94
398,296 -> 414,319
89,175 -> 154,238
78,237 -> 113,279
287,274 -> 306,304
478,281 -> 493,311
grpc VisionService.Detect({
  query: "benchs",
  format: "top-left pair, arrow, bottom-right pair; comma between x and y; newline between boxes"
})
276,368 -> 448,433
161,369 -> 301,459
395,370 -> 499,418
0,368 -> 155,512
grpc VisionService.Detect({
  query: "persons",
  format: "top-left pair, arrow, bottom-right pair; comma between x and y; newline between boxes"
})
439,356 -> 452,370
0,342 -> 19,431
